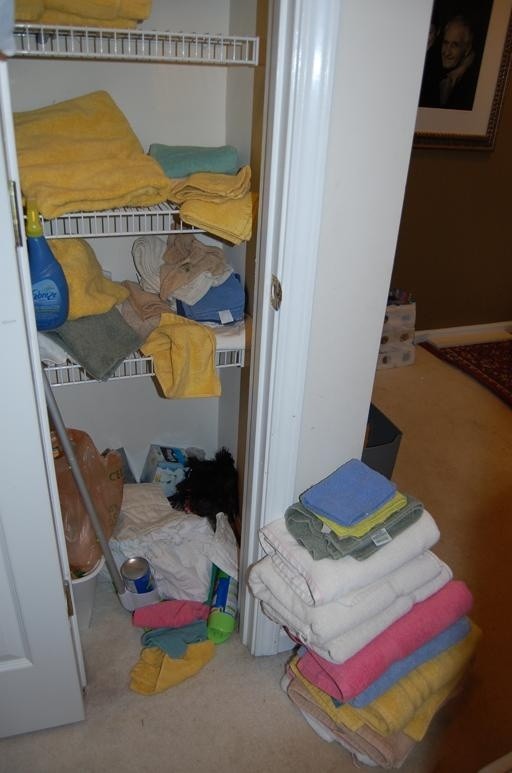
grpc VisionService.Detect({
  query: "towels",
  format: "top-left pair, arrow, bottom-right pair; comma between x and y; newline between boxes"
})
167,165 -> 251,205
130,598 -> 215,697
14,0 -> 153,31
249,458 -> 483,770
148,143 -> 240,178
179,192 -> 253,246
12,90 -> 172,221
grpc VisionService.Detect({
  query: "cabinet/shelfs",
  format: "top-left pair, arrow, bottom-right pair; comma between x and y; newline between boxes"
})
13,23 -> 260,387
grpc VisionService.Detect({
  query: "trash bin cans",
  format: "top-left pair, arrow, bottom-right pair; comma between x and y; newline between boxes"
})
361,402 -> 403,481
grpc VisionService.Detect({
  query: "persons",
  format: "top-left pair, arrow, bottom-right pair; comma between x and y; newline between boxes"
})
424,15 -> 481,111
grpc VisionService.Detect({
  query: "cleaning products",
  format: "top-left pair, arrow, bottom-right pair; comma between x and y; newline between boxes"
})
25,199 -> 69,330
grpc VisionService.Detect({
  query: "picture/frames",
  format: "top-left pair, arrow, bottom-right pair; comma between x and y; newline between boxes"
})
413,0 -> 512,152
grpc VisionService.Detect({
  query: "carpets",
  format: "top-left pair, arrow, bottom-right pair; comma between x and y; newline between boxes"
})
418,324 -> 512,409
